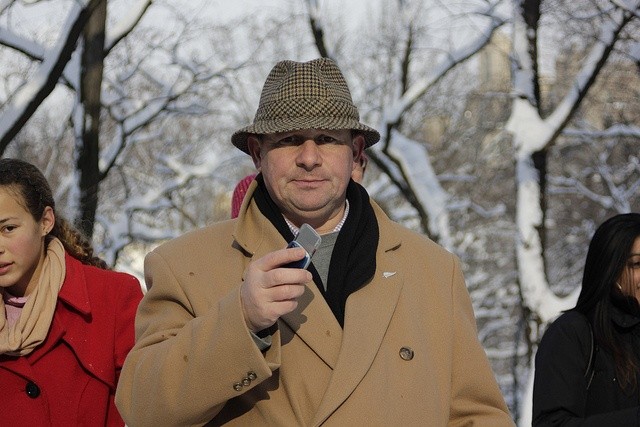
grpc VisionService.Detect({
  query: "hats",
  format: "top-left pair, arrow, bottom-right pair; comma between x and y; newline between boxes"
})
231,57 -> 381,157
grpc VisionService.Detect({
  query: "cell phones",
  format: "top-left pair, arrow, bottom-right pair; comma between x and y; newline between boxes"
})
280,222 -> 322,269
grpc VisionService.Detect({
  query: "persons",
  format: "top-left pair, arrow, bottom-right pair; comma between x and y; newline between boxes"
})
115,56 -> 514,426
531,212 -> 638,426
0,159 -> 144,426
